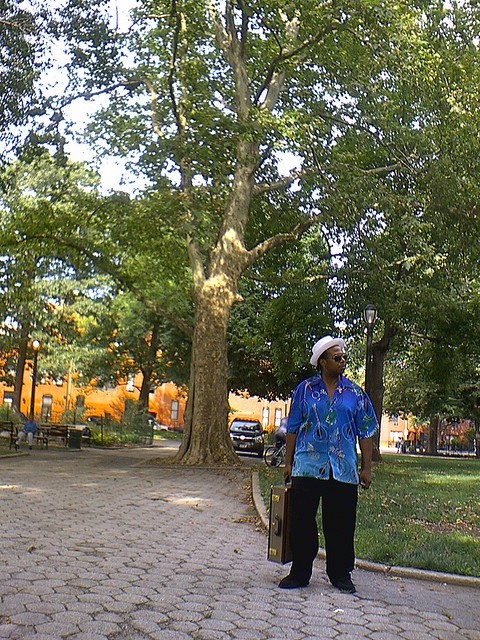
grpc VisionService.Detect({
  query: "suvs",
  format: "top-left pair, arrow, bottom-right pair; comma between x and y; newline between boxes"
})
230,417 -> 265,458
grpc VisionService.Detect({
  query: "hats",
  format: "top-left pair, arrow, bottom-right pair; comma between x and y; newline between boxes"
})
309,335 -> 344,366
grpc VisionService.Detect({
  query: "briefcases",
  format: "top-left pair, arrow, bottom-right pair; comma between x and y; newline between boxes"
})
267,475 -> 293,565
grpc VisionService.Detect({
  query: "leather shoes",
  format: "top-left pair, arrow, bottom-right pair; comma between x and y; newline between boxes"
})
279,573 -> 309,588
335,579 -> 356,593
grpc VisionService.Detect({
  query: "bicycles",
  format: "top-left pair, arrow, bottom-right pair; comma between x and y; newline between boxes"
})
265,441 -> 287,467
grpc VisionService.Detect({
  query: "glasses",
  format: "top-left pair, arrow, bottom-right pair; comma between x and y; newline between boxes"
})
322,353 -> 348,362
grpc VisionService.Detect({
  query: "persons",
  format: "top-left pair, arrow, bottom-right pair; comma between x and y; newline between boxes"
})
271,417 -> 288,467
14,415 -> 38,450
279,334 -> 380,595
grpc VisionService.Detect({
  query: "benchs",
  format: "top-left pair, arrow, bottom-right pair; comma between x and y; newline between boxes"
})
41,426 -> 68,445
0,422 -> 20,452
15,424 -> 51,449
